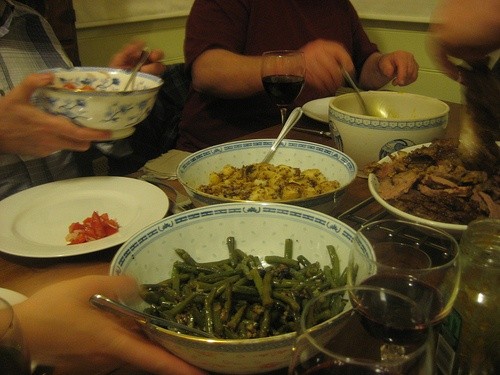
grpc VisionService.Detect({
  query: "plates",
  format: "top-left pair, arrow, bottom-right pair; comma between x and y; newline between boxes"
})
301,97 -> 332,124
1,288 -> 37,375
0,176 -> 171,257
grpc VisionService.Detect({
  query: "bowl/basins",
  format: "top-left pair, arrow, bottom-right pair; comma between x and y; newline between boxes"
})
110,202 -> 377,375
368,143 -> 500,239
177,139 -> 358,216
36,67 -> 164,141
327,91 -> 449,179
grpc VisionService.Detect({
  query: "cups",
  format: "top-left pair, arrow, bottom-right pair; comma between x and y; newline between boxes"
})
288,285 -> 433,375
1,298 -> 31,375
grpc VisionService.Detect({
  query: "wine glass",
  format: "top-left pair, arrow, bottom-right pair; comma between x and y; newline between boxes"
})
261,50 -> 306,129
453,219 -> 499,375
346,217 -> 461,375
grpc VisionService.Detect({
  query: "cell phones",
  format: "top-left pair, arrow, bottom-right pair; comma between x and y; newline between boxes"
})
294,118 -> 328,134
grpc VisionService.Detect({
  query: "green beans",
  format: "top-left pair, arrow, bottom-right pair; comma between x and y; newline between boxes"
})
138,236 -> 358,339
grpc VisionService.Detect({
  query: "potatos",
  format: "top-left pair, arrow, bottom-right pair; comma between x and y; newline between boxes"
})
202,165 -> 342,202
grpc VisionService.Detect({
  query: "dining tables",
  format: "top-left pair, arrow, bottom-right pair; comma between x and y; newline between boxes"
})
0,102 -> 500,375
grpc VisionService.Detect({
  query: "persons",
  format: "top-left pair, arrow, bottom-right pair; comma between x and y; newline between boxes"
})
0,1 -> 167,206
427,0 -> 500,81
174,0 -> 420,153
0,274 -> 211,374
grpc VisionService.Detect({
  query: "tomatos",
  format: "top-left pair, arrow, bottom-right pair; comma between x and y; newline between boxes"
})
68,210 -> 119,244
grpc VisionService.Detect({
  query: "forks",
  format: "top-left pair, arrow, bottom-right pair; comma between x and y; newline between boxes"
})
142,174 -> 197,211
